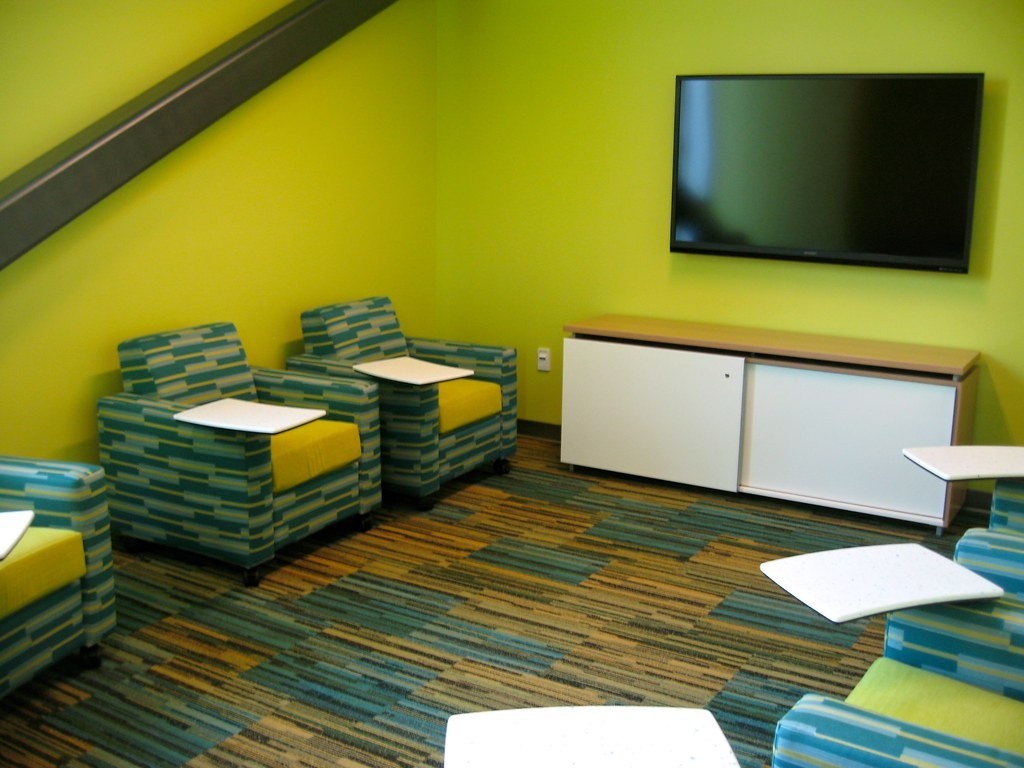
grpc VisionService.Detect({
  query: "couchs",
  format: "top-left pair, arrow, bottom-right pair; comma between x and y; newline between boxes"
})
952,480 -> 1024,606
0,456 -> 116,722
286,296 -> 516,504
95,322 -> 382,585
769,603 -> 1024,768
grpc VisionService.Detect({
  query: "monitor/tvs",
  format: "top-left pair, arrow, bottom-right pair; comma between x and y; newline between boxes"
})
670,73 -> 984,275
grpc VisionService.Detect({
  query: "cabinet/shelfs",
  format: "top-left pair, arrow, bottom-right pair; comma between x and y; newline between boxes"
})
562,314 -> 980,539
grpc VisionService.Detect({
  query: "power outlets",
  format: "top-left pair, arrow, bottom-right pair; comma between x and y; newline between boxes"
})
537,347 -> 551,372
